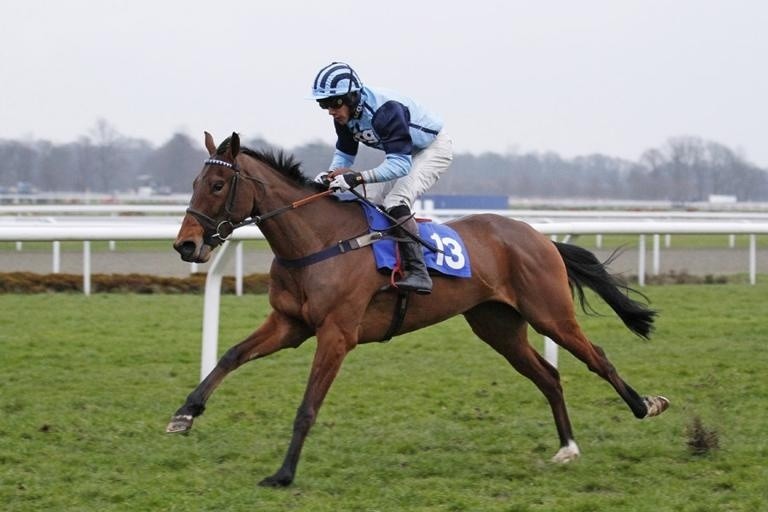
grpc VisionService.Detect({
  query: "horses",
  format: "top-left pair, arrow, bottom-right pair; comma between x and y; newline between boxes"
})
165,128 -> 672,489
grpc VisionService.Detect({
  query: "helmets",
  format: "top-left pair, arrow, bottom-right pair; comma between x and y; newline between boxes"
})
312,61 -> 363,101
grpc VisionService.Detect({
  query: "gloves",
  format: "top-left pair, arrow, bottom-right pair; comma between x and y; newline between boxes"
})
328,172 -> 364,194
314,171 -> 334,184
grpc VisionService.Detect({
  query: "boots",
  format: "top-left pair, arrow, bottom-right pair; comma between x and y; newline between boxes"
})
394,214 -> 433,295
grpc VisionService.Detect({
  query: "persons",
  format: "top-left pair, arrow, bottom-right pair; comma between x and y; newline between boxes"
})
305,62 -> 453,295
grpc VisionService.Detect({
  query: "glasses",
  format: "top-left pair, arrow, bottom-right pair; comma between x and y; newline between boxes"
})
319,97 -> 344,109
